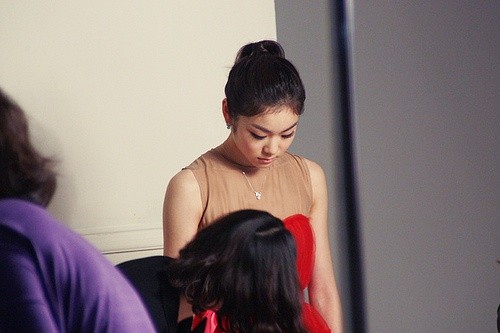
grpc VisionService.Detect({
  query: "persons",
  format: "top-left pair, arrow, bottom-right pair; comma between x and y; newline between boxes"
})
0,86 -> 160,333
113,209 -> 306,332
163,41 -> 343,333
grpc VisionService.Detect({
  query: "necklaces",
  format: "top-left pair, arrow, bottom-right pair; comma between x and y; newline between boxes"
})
221,141 -> 269,201
